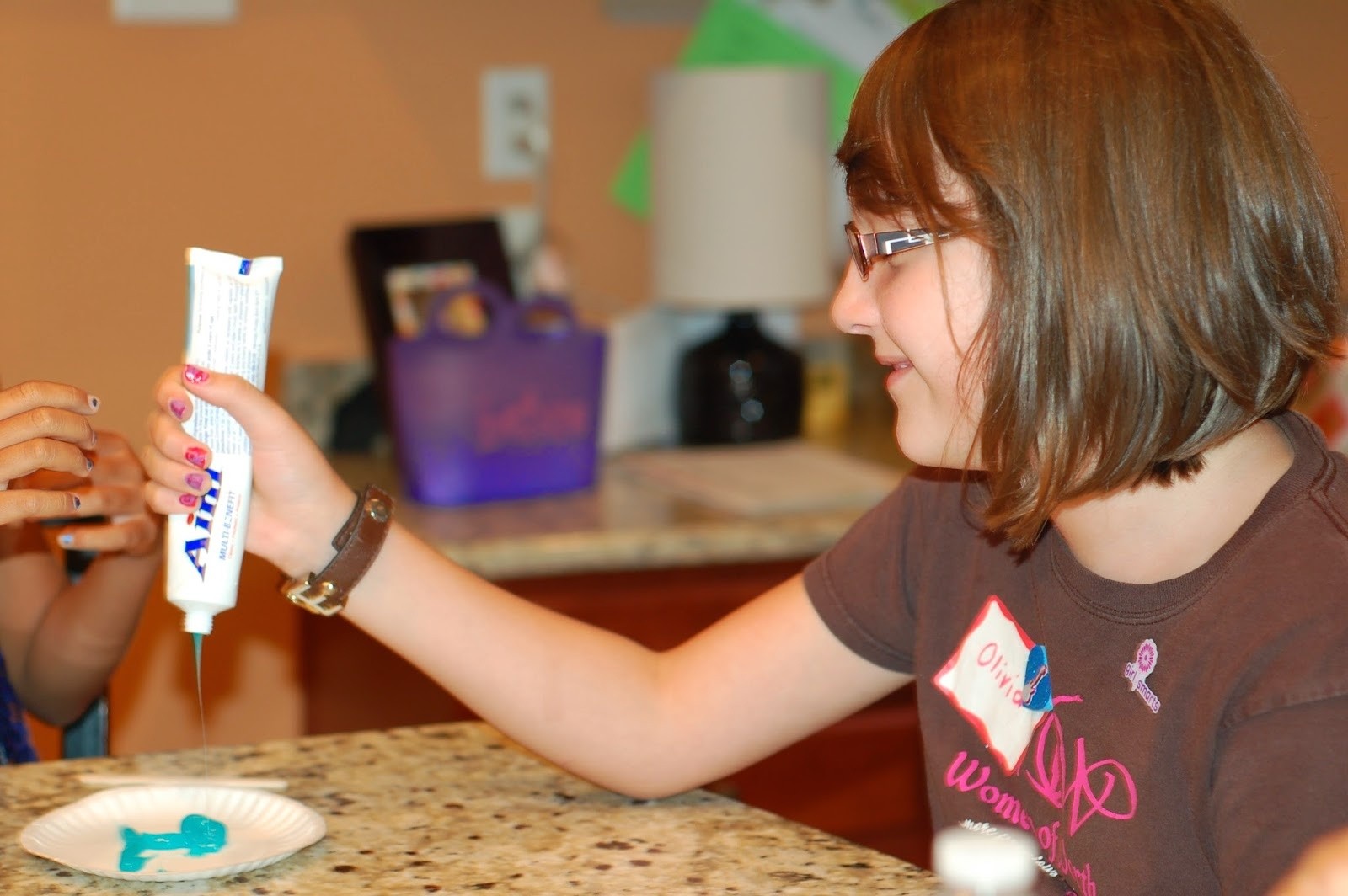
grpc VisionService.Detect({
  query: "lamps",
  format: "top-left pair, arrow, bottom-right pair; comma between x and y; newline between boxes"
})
650,62 -> 838,445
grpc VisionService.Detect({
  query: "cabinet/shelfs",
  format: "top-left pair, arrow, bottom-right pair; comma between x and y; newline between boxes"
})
303,438 -> 940,875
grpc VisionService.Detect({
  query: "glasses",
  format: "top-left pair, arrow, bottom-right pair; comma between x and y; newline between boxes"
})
845,220 -> 983,281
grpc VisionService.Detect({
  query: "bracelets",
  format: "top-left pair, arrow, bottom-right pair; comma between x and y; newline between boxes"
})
278,484 -> 394,618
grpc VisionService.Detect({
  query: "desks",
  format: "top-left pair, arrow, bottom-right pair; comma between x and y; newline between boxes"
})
2,715 -> 953,896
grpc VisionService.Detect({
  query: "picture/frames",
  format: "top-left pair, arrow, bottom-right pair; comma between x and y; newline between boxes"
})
325,212 -> 519,450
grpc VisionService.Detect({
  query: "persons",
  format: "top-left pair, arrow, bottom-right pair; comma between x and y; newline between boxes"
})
1,380 -> 161,768
138,0 -> 1347,890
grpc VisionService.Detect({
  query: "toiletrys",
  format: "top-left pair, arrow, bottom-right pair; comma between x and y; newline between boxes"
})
162,245 -> 288,635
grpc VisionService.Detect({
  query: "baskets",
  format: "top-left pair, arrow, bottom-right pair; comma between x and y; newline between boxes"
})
391,284 -> 604,507
